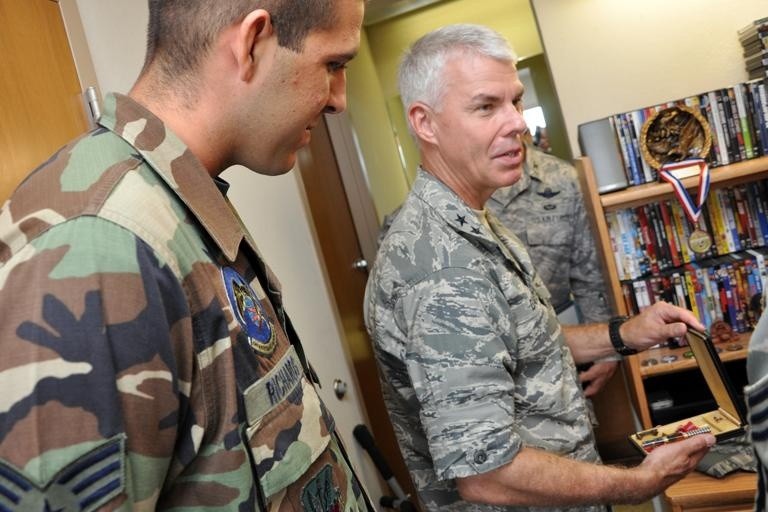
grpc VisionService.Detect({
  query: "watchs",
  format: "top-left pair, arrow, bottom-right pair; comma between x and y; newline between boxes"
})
608,315 -> 644,355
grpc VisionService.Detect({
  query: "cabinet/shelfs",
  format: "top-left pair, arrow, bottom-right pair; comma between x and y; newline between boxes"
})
574,154 -> 766,512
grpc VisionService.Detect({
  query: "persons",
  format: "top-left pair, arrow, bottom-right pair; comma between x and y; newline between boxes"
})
0,0 -> 366,512
362,22 -> 716,512
376,139 -> 624,428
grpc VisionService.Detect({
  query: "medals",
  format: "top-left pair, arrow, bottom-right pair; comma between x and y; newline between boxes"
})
656,157 -> 713,254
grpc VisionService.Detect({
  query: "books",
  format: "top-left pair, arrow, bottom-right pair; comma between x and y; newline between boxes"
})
738,16 -> 768,81
609,81 -> 768,186
604,178 -> 768,281
619,246 -> 768,350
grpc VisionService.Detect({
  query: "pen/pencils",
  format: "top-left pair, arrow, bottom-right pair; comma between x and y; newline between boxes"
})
641,426 -> 712,449
